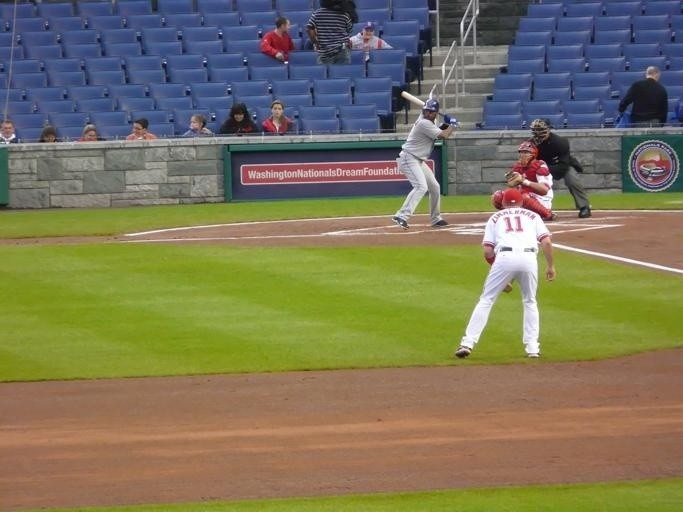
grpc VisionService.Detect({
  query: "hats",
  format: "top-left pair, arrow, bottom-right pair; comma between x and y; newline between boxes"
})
502,189 -> 523,209
423,99 -> 440,112
363,21 -> 375,31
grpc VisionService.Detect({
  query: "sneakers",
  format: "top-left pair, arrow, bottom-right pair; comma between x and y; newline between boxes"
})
392,216 -> 409,230
551,212 -> 557,220
434,220 -> 449,228
454,346 -> 471,357
578,208 -> 591,218
528,353 -> 540,358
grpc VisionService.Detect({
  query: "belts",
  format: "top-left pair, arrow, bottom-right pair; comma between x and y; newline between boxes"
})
500,247 -> 535,252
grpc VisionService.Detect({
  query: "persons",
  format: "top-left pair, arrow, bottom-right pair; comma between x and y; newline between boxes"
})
37,125 -> 58,142
614,65 -> 669,126
179,113 -> 215,138
258,0 -> 394,65
527,116 -> 590,218
385,98 -> 464,232
78,123 -> 97,141
124,118 -> 156,140
453,187 -> 556,359
0,119 -> 22,143
499,140 -> 554,223
217,102 -> 261,134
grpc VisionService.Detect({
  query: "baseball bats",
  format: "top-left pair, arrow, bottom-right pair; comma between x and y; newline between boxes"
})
401,91 -> 461,127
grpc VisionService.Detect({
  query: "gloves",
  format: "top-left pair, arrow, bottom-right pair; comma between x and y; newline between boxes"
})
450,118 -> 457,128
443,115 -> 451,126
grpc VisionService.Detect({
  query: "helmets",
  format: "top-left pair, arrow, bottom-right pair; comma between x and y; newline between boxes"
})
516,141 -> 538,167
492,190 -> 505,210
530,119 -> 549,145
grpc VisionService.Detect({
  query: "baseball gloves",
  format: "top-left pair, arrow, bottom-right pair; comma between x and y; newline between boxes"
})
504,172 -> 523,188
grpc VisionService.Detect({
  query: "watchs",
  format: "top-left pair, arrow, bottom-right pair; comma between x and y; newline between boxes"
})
260,100 -> 293,134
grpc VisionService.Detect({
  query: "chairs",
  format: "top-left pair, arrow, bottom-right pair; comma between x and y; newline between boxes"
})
475,3 -> 681,128
2,1 -> 436,135
